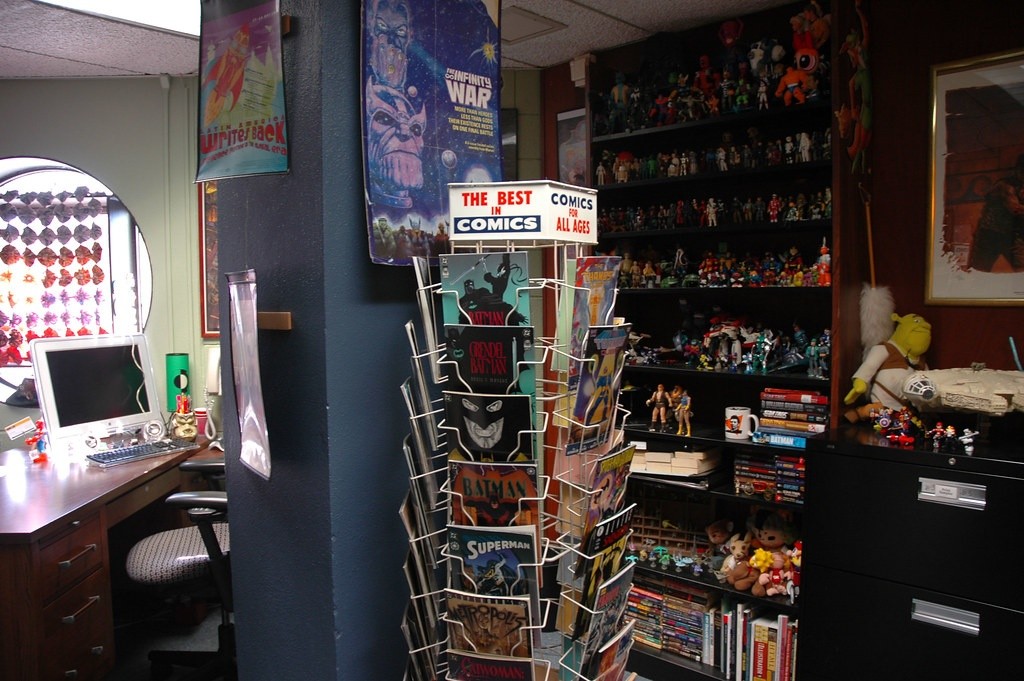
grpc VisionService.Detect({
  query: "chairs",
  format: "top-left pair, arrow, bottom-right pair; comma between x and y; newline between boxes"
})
130,459 -> 238,681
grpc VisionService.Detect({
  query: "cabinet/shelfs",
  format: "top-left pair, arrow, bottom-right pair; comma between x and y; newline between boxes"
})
581,0 -> 875,681
794,409 -> 1024,681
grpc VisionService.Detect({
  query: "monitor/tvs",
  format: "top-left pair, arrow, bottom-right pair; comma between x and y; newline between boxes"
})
29,332 -> 161,451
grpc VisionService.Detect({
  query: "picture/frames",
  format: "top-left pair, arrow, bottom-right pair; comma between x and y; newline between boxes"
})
197,181 -> 220,339
926,51 -> 1024,306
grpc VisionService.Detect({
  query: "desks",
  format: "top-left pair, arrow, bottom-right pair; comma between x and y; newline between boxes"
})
0,429 -> 214,681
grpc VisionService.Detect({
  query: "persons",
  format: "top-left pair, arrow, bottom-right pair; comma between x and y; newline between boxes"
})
645,384 -> 696,436
590,0 -> 832,376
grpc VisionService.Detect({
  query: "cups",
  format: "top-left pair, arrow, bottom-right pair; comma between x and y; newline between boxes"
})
175,412 -> 194,438
194,408 -> 212,436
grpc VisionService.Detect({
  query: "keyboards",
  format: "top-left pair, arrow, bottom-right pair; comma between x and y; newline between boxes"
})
85,437 -> 200,467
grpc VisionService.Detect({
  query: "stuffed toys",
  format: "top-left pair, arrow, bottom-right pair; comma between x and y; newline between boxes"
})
846,310 -> 933,442
705,508 -> 804,603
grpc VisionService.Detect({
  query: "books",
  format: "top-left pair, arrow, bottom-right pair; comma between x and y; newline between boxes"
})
752,389 -> 829,449
733,456 -> 805,505
399,251 -> 636,681
623,576 -> 798,681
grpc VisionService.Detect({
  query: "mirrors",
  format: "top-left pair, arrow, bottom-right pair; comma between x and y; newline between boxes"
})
0,155 -> 156,409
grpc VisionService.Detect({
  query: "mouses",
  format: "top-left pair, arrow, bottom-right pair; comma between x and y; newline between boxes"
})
208,438 -> 224,451
